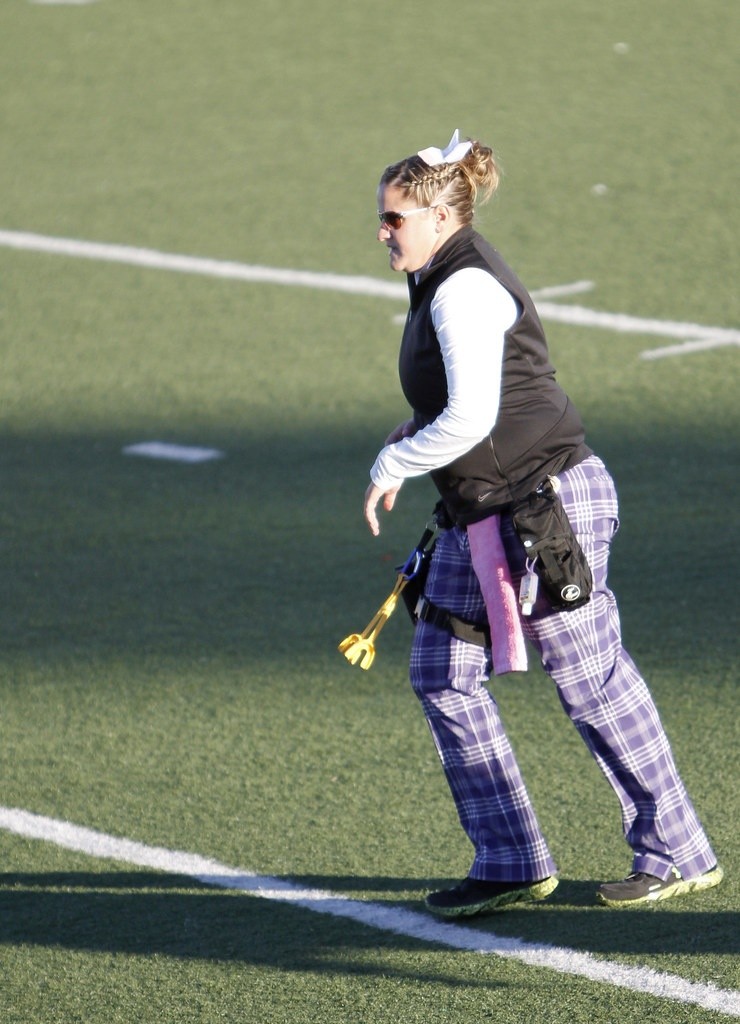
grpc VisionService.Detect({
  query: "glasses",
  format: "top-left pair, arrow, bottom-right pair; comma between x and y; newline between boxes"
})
376,207 -> 426,231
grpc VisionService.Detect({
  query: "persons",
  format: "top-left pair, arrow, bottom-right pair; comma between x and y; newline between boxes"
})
364,128 -> 724,915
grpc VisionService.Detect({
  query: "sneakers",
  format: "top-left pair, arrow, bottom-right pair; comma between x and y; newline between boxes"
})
423,873 -> 558,916
596,860 -> 725,908
